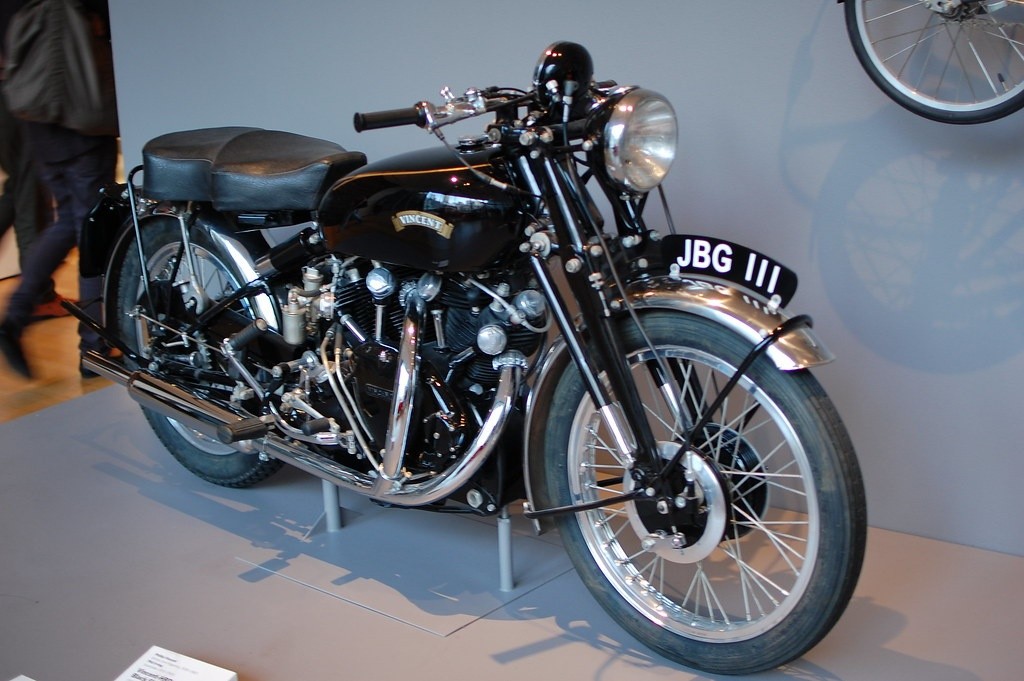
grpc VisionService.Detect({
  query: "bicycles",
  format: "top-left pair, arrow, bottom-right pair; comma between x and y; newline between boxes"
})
835,0 -> 1023,125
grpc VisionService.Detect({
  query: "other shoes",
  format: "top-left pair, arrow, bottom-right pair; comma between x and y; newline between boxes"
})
31,294 -> 77,317
80,356 -> 124,378
0,326 -> 30,376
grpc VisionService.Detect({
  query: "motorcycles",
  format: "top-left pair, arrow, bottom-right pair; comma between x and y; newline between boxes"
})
57,40 -> 870,678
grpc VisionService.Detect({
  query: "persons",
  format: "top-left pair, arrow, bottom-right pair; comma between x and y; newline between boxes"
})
0,0 -> 120,380
